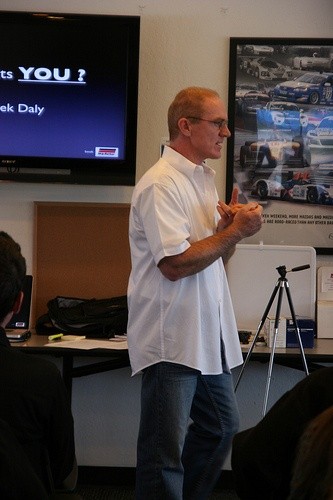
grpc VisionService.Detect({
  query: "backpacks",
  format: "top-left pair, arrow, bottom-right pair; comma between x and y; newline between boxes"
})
36,295 -> 128,341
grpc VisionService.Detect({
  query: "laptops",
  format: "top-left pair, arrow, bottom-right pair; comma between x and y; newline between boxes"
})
2,274 -> 34,338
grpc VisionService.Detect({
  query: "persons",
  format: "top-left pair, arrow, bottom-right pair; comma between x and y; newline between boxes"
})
292,403 -> 333,499
1,228 -> 81,500
126,84 -> 264,500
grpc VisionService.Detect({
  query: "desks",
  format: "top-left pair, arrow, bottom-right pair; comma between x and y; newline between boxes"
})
4,327 -> 333,411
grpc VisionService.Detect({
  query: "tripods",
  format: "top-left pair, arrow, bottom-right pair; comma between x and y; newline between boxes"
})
235,263 -> 312,421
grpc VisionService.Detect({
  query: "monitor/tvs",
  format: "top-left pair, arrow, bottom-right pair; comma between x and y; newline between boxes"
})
0,10 -> 140,187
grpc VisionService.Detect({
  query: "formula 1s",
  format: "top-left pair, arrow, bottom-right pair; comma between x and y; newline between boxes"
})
249,166 -> 333,205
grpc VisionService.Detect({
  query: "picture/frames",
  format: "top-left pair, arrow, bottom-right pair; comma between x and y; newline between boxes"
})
225,36 -> 333,257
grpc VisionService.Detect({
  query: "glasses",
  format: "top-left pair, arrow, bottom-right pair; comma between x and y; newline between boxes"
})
183,116 -> 229,129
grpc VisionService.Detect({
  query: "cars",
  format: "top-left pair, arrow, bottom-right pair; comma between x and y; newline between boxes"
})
264,102 -> 314,140
236,90 -> 270,117
242,44 -> 275,56
239,56 -> 292,82
306,137 -> 333,171
289,45 -> 330,73
239,138 -> 312,170
276,71 -> 333,105
299,108 -> 333,137
